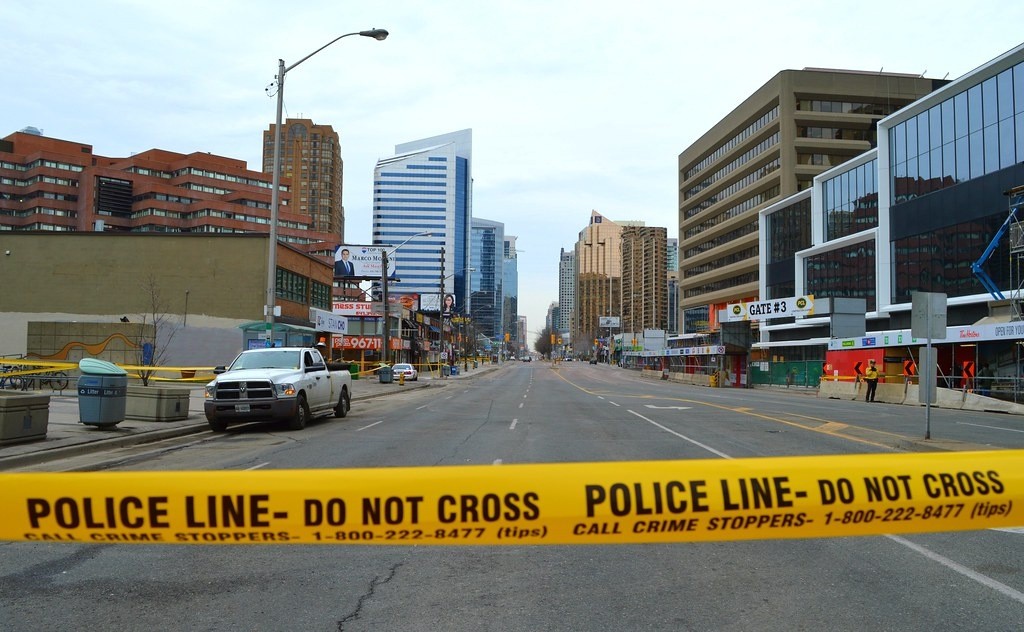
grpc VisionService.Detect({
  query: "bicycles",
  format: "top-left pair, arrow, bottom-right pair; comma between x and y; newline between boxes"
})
0,353 -> 69,392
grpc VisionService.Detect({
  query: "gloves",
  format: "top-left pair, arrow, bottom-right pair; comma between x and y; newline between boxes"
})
871,367 -> 875,371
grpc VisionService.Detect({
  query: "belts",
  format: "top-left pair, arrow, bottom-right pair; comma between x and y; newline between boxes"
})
869,379 -> 877,382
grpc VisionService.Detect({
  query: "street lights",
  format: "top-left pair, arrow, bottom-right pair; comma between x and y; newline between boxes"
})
606,309 -> 625,369
437,267 -> 477,369
380,231 -> 433,365
463,291 -> 486,372
266,27 -> 390,347
473,304 -> 491,361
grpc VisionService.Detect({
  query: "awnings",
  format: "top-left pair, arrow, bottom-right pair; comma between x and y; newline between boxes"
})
405,320 -> 421,328
429,326 -> 440,332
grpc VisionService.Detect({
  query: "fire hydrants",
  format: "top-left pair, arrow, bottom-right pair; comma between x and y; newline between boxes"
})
397,372 -> 405,386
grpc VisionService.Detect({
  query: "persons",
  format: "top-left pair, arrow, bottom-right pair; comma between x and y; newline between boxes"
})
978,363 -> 996,396
443,294 -> 454,311
120,317 -> 129,322
335,249 -> 355,276
414,339 -> 418,345
866,359 -> 880,403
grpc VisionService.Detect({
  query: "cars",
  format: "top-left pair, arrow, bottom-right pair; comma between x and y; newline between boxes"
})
509,355 -> 599,364
391,362 -> 418,381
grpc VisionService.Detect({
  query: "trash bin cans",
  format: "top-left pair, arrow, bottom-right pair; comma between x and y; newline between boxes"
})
379,363 -> 391,383
444,365 -> 450,376
78,358 -> 128,430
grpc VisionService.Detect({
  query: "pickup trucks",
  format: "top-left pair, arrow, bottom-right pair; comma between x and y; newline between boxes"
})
204,348 -> 352,432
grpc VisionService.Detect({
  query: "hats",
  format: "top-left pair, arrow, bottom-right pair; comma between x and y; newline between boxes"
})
870,360 -> 876,364
